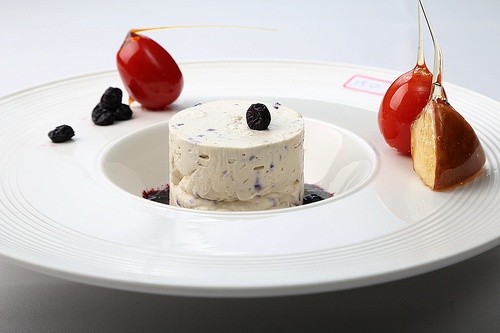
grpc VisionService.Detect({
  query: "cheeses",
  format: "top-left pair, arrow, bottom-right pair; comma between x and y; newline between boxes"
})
168,100 -> 305,212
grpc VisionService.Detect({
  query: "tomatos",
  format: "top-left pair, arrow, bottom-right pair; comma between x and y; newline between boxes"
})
116,32 -> 184,109
377,66 -> 444,156
